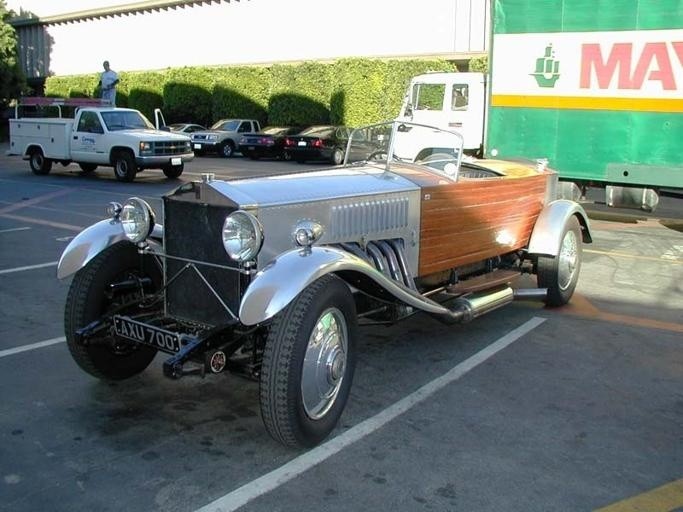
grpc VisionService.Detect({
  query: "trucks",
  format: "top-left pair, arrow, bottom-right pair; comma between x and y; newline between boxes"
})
387,0 -> 682,214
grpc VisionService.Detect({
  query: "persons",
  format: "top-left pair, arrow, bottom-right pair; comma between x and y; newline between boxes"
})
96,60 -> 118,105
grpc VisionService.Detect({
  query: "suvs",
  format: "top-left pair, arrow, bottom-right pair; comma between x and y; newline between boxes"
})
190,119 -> 260,157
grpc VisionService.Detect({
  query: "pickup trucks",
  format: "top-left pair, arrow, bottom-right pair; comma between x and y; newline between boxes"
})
5,98 -> 195,180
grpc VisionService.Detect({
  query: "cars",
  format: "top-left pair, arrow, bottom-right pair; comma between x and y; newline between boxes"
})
167,124 -> 208,138
240,126 -> 302,161
56,120 -> 591,447
286,126 -> 366,164
